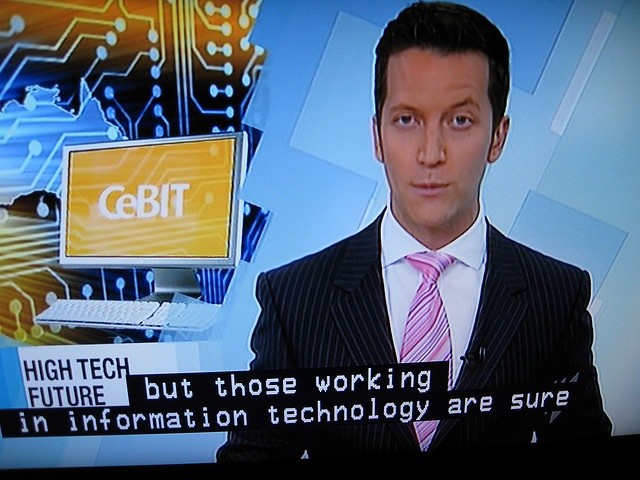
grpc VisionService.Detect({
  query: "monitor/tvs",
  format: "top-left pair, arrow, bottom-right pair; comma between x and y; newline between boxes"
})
60,131 -> 248,303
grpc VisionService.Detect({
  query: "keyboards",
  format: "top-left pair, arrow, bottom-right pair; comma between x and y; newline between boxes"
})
34,299 -> 223,332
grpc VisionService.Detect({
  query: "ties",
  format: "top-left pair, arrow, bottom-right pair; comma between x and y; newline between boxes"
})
400,252 -> 455,451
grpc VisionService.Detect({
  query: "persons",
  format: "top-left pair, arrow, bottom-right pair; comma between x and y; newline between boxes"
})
217,1 -> 614,465
